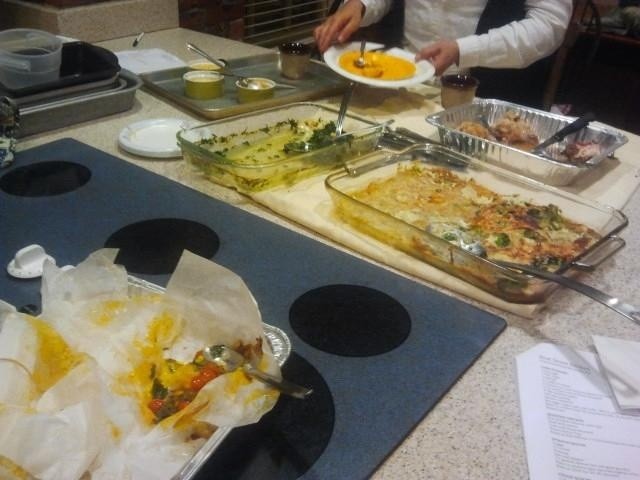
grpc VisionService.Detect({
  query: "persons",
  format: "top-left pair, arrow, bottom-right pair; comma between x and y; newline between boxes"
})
313,0 -> 573,107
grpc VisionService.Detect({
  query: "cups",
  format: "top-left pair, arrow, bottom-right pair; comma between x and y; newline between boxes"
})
181,59 -> 225,101
235,75 -> 274,104
440,72 -> 481,108
277,40 -> 310,80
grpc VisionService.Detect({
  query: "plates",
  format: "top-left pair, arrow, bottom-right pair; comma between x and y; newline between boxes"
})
320,40 -> 437,89
120,118 -> 209,160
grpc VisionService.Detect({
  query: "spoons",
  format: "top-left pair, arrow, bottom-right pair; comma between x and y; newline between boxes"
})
284,119 -> 395,153
203,346 -> 316,400
354,31 -> 369,70
